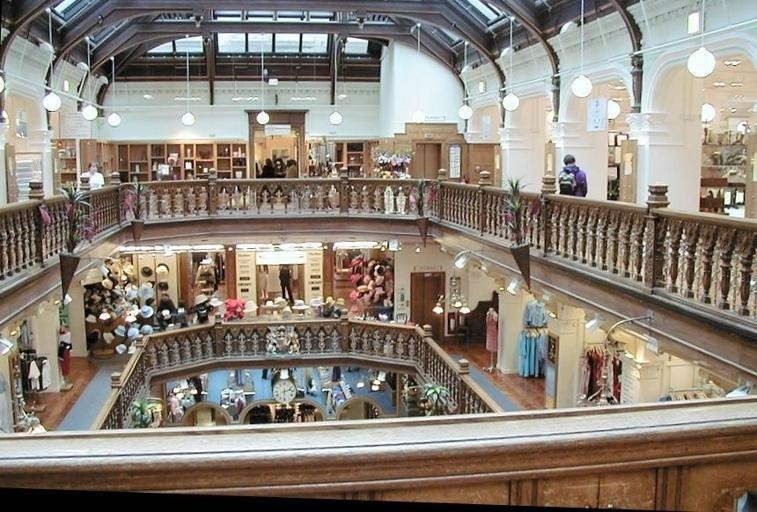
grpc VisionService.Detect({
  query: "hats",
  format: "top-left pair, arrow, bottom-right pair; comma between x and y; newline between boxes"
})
350,257 -> 391,300
86,262 -> 171,355
193,293 -> 344,313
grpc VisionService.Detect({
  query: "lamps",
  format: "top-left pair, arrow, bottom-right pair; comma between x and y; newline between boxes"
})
508,281 -> 520,295
131,222 -> 144,241
454,251 -> 470,269
415,218 -> 428,247
432,273 -> 471,314
32,0 -> 619,127
585,315 -> 601,333
511,246 -> 530,290
60,256 -> 80,299
688,0 -> 716,78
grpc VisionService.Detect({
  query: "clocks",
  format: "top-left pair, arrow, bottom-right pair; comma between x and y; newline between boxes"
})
271,379 -> 298,405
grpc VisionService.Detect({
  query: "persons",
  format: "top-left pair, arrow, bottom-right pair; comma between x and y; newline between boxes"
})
278,266 -> 296,304
557,155 -> 587,198
258,159 -> 273,177
483,307 -> 498,353
283,160 -> 297,178
156,292 -> 175,313
79,162 -> 106,190
259,265 -> 269,299
140,298 -> 154,327
607,152 -> 620,182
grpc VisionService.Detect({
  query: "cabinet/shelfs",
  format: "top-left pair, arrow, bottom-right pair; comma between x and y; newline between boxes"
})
295,142 -> 377,180
52,140 -> 246,187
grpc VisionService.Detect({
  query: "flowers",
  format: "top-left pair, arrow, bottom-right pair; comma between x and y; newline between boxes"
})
496,179 -> 543,246
405,181 -> 439,216
373,152 -> 411,178
122,184 -> 151,219
38,189 -> 100,252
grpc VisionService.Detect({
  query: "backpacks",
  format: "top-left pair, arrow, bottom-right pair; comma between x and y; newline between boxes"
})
558,170 -> 576,195
